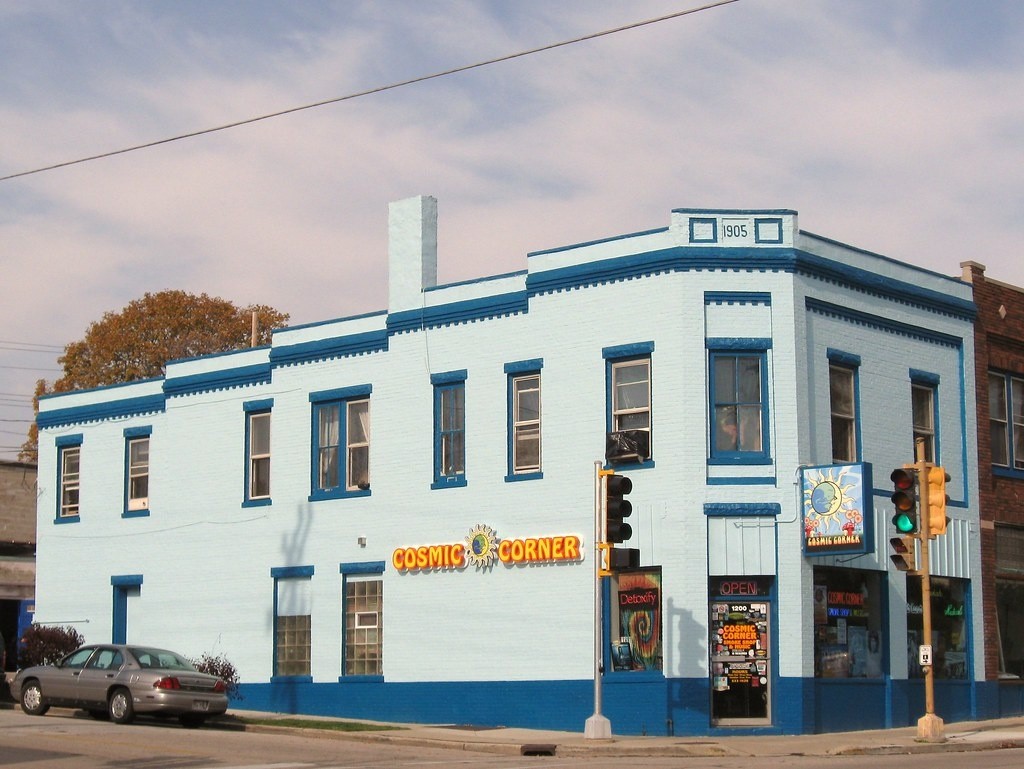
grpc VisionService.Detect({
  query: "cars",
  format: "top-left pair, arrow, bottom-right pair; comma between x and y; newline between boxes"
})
10,644 -> 228,729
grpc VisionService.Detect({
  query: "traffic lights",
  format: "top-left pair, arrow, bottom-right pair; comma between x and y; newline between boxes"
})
606,473 -> 633,544
890,468 -> 918,533
604,546 -> 641,577
890,536 -> 916,571
